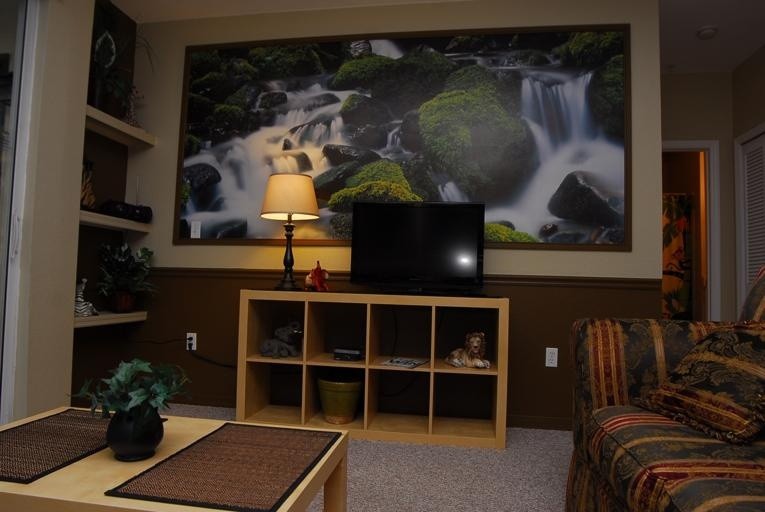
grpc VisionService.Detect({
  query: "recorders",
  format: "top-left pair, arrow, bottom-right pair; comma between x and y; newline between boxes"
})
101,199 -> 152,223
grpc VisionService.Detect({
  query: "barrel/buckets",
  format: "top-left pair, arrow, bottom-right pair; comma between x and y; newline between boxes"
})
317,378 -> 361,425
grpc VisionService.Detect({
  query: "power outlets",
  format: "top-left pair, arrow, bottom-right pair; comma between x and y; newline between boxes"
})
544,347 -> 560,368
185,330 -> 199,351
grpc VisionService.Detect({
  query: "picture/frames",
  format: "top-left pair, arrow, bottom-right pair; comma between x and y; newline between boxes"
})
171,19 -> 633,252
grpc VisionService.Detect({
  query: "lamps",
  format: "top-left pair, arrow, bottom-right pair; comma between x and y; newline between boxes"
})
259,171 -> 322,290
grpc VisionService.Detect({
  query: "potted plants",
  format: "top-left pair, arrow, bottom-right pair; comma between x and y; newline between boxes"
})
95,246 -> 158,315
71,361 -> 197,464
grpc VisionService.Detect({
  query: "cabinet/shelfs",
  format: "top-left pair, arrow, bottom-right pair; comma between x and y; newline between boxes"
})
65,0 -> 161,332
230,285 -> 514,453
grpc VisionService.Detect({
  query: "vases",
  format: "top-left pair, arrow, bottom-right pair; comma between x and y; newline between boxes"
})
314,376 -> 362,427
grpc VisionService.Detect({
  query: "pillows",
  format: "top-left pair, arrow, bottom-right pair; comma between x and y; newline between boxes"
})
632,322 -> 765,446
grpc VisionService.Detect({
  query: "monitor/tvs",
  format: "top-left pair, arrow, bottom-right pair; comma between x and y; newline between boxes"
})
350,201 -> 485,297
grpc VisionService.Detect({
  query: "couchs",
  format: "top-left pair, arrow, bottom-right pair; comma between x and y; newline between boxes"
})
565,260 -> 765,511
